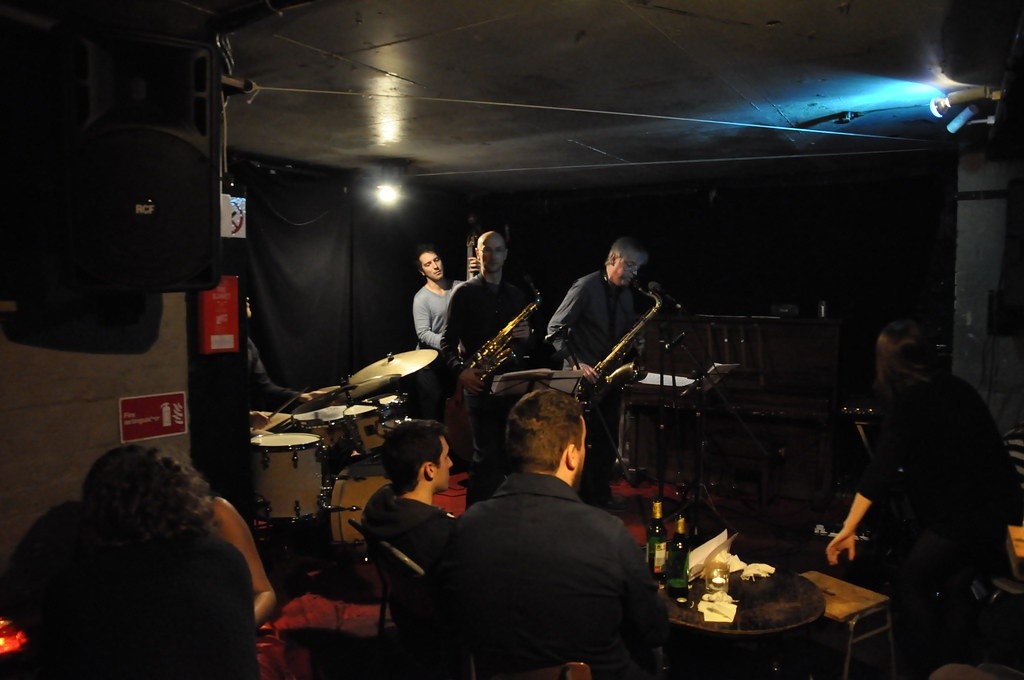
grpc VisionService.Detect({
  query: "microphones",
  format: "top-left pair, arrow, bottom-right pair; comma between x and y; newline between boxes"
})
543,325 -> 566,345
647,282 -> 682,310
664,331 -> 687,352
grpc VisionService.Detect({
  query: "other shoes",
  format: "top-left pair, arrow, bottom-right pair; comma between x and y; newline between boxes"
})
592,501 -> 630,512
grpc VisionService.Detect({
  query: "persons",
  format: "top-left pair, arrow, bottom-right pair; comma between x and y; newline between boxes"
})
440,230 -> 543,501
546,238 -> 649,509
189,297 -> 340,527
411,249 -> 481,420
0,444 -> 276,680
825,318 -> 1021,680
361,419 -> 456,568
431,390 -> 670,680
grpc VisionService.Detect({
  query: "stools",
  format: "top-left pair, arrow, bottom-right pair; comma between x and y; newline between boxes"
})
800,523 -> 1024,680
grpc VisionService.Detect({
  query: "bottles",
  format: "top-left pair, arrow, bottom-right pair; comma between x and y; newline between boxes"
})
664,512 -> 689,600
645,499 -> 667,584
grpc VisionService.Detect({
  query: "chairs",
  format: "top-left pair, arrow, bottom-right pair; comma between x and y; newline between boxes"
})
348,519 -> 592,680
28,535 -> 263,680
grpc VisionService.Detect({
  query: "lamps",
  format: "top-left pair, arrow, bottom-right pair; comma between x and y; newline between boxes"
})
930,86 -> 991,117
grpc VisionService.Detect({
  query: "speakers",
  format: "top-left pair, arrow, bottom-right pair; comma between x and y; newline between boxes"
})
50,21 -> 222,295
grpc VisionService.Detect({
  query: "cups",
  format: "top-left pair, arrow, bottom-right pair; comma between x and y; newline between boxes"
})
705,562 -> 730,594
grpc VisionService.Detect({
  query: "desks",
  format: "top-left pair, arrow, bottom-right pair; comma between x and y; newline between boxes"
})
659,572 -> 826,680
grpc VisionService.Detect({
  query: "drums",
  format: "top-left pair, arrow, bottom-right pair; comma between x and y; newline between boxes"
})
249,433 -> 327,521
329,461 -> 394,545
344,394 -> 410,456
291,396 -> 347,454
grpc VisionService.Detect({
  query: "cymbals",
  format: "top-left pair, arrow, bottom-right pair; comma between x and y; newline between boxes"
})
345,348 -> 439,399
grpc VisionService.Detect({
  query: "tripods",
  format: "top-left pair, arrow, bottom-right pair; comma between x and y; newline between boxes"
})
657,344 -> 769,548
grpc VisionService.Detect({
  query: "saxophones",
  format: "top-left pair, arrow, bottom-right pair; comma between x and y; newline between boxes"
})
468,281 -> 543,380
574,279 -> 664,413
